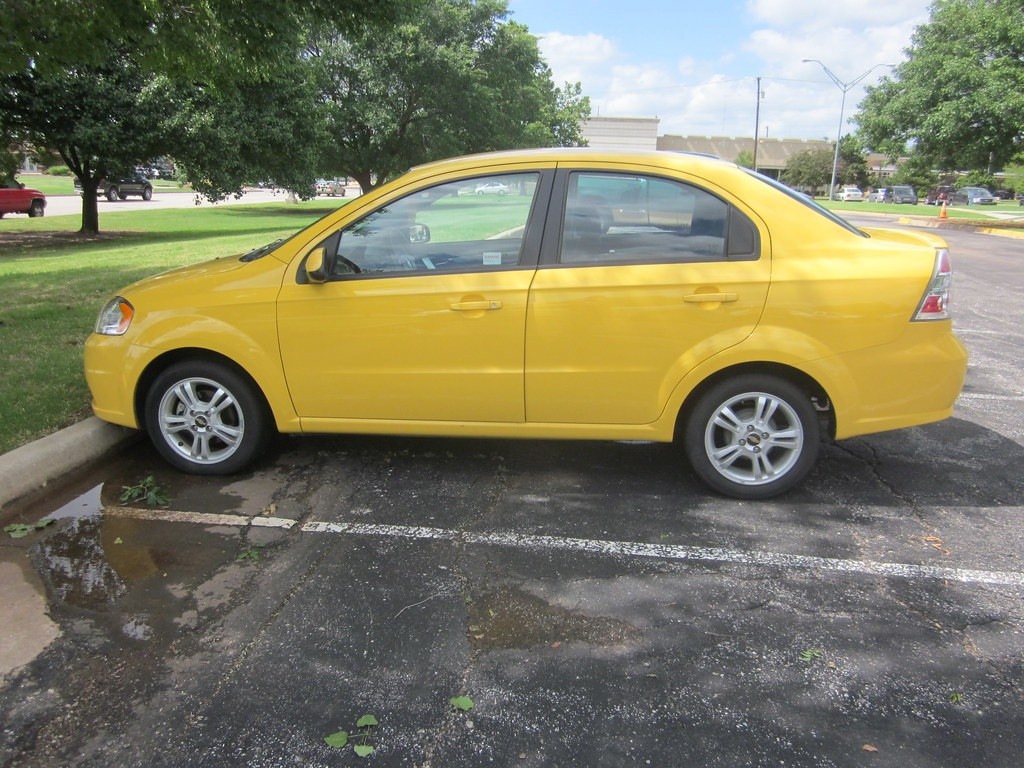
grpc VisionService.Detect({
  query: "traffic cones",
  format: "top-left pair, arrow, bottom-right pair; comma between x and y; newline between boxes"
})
938,200 -> 948,218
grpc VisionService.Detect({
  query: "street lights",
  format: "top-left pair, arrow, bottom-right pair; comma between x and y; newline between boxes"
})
802,56 -> 895,204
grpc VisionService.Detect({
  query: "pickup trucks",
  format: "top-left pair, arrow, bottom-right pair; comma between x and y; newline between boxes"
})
924,185 -> 968,205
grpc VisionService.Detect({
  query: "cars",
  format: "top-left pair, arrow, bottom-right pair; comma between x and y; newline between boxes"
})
316,181 -> 346,196
475,181 -> 509,196
958,187 -> 998,205
834,186 -> 866,205
0,174 -> 48,219
74,167 -> 154,202
884,185 -> 919,205
868,189 -> 887,203
79,146 -> 974,498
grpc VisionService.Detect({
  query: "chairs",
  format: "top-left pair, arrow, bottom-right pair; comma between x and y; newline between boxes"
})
565,192 -> 617,253
